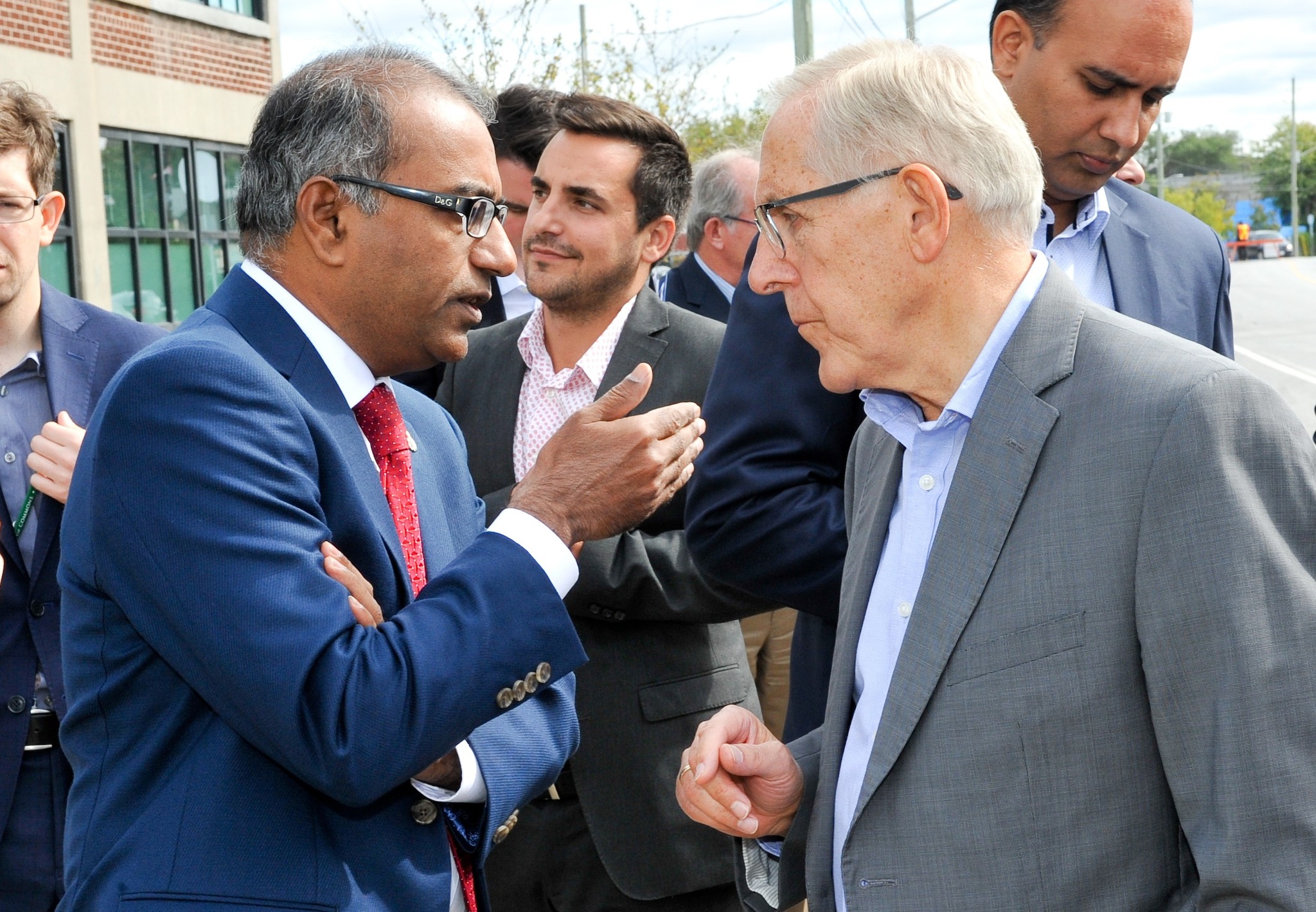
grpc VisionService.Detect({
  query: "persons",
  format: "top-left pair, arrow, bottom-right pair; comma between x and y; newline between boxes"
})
683,0 -> 1236,912
392,44 -> 1316,912
431,94 -> 765,912
0,81 -> 192,912
1237,220 -> 1250,260
58,43 -> 707,912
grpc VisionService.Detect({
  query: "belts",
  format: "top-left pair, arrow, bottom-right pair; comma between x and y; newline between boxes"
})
20,708 -> 61,751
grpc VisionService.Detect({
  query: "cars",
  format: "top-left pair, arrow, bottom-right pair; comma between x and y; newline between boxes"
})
1242,229 -> 1293,259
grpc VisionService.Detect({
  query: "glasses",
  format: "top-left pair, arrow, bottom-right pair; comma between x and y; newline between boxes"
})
754,168 -> 963,260
325,174 -> 508,238
0,192 -> 46,225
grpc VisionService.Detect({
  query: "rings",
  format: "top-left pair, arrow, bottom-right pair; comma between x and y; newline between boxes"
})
679,766 -> 691,785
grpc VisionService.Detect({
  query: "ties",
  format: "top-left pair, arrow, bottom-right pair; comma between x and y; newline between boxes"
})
351,385 -> 477,911
